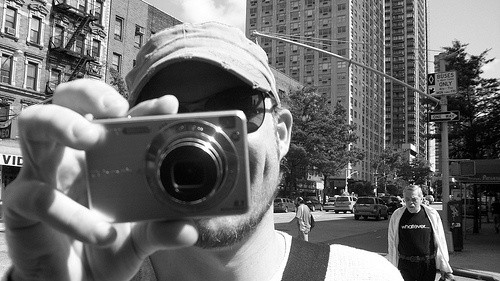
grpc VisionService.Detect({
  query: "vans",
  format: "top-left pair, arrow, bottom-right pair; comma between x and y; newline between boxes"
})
274,198 -> 296,212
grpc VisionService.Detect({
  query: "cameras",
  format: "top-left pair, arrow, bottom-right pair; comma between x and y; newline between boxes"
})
80,110 -> 251,224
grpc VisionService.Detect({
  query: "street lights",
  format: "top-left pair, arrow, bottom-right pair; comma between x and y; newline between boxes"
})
2,51 -> 23,66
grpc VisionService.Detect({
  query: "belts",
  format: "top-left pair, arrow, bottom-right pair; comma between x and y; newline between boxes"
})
398,253 -> 436,262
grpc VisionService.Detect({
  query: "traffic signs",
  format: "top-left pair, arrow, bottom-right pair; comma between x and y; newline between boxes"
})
428,111 -> 460,121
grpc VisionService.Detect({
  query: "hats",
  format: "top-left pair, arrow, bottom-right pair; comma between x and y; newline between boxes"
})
124,19 -> 279,106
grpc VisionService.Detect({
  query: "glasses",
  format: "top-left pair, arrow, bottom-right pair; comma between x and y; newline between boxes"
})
157,88 -> 266,134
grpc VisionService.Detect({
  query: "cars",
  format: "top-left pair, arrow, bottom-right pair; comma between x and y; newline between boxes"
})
323,197 -> 335,212
304,198 -> 323,211
354,197 -> 390,220
381,196 -> 403,207
334,196 -> 358,213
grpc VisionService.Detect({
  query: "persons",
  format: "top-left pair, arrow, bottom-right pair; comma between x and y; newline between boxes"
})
387,185 -> 456,281
288,197 -> 312,241
0,22 -> 405,281
